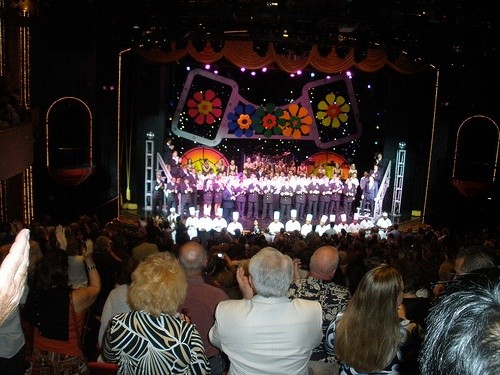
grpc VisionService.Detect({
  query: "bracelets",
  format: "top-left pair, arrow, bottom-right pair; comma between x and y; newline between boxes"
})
86,264 -> 97,271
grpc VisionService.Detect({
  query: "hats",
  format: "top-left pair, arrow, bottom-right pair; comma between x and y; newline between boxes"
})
382,212 -> 388,216
233,211 -> 239,219
306,214 -> 313,221
290,209 -> 297,218
169,207 -> 175,212
274,210 -> 280,219
341,214 -> 346,222
364,214 -> 369,217
354,213 -> 358,220
189,207 -> 195,215
217,208 -> 223,215
204,205 -> 210,215
320,214 -> 328,223
330,214 -> 335,222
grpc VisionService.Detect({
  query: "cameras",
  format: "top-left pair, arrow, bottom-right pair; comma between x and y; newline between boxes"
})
216,252 -> 224,259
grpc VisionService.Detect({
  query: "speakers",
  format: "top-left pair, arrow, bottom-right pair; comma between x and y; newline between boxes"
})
47,96 -> 94,184
451,115 -> 500,194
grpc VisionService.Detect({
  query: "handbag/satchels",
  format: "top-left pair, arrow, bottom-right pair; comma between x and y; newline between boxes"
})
69,285 -> 102,361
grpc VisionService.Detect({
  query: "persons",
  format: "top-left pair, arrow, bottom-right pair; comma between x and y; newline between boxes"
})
176,241 -> 229,375
0,152 -> 500,288
96,241 -> 158,364
417,279 -> 498,375
326,264 -> 423,375
288,246 -> 350,375
0,229 -> 30,325
105,252 -> 211,375
208,246 -> 323,375
24,225 -> 101,375
0,306 -> 26,375
